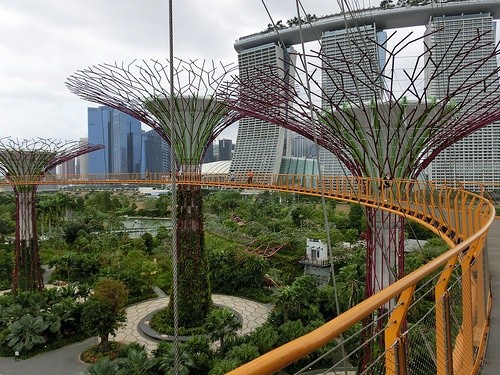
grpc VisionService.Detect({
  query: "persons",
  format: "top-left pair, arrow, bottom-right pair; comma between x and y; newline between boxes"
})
172,169 -> 182,181
230,171 -> 235,181
247,169 -> 254,184
382,171 -> 393,203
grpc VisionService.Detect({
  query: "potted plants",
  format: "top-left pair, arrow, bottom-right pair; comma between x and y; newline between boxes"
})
78,277 -> 130,375
